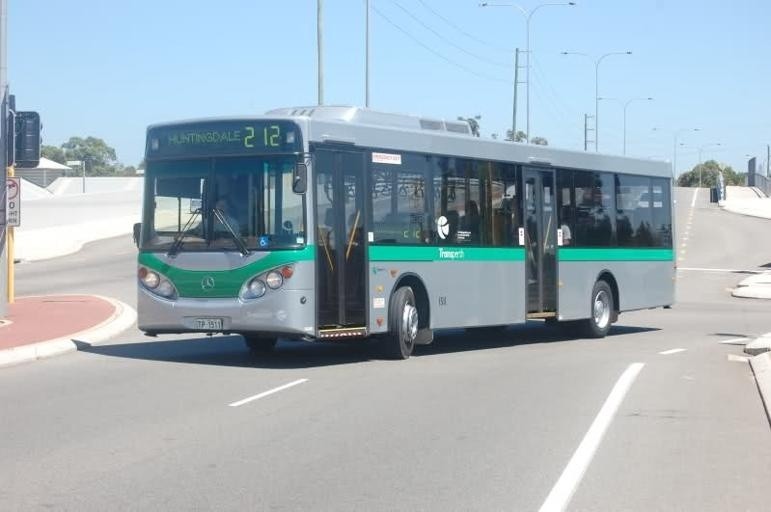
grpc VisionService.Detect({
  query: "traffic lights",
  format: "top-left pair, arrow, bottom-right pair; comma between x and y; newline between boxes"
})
14,110 -> 46,168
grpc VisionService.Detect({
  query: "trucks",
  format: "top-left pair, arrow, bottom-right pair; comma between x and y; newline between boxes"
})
189,194 -> 203,213
498,182 -> 552,212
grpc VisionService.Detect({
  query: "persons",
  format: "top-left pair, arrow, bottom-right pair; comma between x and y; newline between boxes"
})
196,194 -> 240,239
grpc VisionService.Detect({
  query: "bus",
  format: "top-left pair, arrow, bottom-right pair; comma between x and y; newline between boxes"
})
129,103 -> 678,362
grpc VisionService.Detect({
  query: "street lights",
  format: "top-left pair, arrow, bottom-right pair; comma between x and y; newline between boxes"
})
597,94 -> 653,155
652,123 -> 701,189
479,0 -> 577,143
559,48 -> 633,154
679,138 -> 721,185
745,152 -> 770,182
65,159 -> 87,193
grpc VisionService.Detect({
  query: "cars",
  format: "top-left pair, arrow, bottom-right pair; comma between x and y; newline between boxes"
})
633,188 -> 663,212
